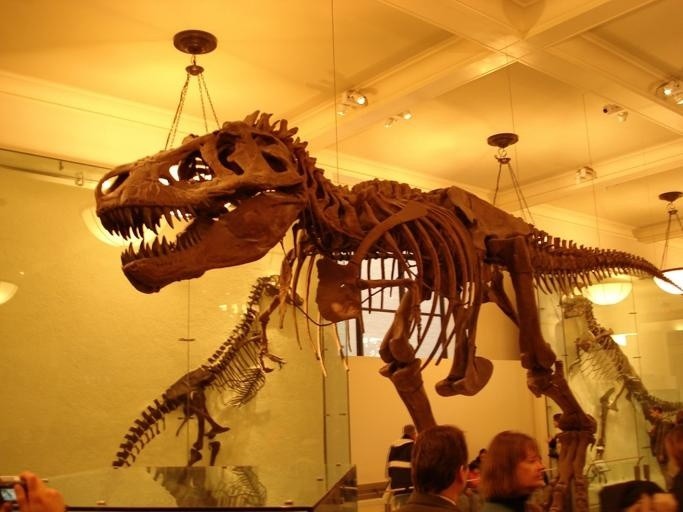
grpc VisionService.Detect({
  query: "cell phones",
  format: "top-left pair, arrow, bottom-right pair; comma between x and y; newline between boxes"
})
0,474 -> 27,507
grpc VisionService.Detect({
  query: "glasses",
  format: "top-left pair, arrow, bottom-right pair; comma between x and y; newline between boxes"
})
653,189 -> 683,294
569,94 -> 634,306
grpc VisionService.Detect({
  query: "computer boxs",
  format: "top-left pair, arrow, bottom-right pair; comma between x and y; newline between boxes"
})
603,103 -> 621,115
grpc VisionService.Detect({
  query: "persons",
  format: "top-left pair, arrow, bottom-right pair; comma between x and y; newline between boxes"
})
0,471 -> 65,512
386,407 -> 683,512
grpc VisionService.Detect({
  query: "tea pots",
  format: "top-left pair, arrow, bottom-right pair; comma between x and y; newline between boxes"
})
0,147 -> 352,512
